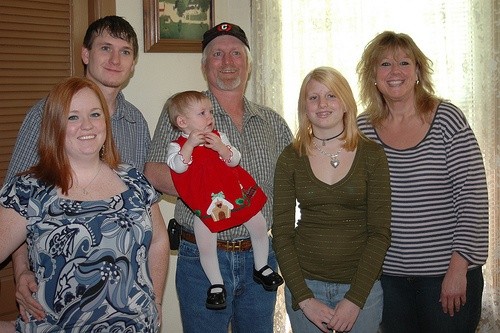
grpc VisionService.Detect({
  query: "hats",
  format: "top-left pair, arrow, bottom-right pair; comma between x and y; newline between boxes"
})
200,21 -> 251,53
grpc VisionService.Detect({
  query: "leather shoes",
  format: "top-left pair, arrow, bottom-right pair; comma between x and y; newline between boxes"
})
252,264 -> 284,290
206,281 -> 228,310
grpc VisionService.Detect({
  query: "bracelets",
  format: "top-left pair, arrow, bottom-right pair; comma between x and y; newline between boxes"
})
301,297 -> 311,310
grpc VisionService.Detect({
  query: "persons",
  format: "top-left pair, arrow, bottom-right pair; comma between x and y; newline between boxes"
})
164,91 -> 285,309
143,22 -> 296,333
271,66 -> 392,333
0,77 -> 171,333
3,16 -> 152,323
357,31 -> 489,333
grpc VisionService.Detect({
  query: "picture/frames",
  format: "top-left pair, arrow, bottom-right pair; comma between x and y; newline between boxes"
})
143,0 -> 215,53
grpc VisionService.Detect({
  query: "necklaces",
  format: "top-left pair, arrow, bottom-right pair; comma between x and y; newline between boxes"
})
311,139 -> 346,168
312,129 -> 344,146
74,165 -> 102,194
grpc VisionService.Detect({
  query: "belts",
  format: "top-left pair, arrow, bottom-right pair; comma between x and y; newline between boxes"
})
178,229 -> 252,253
